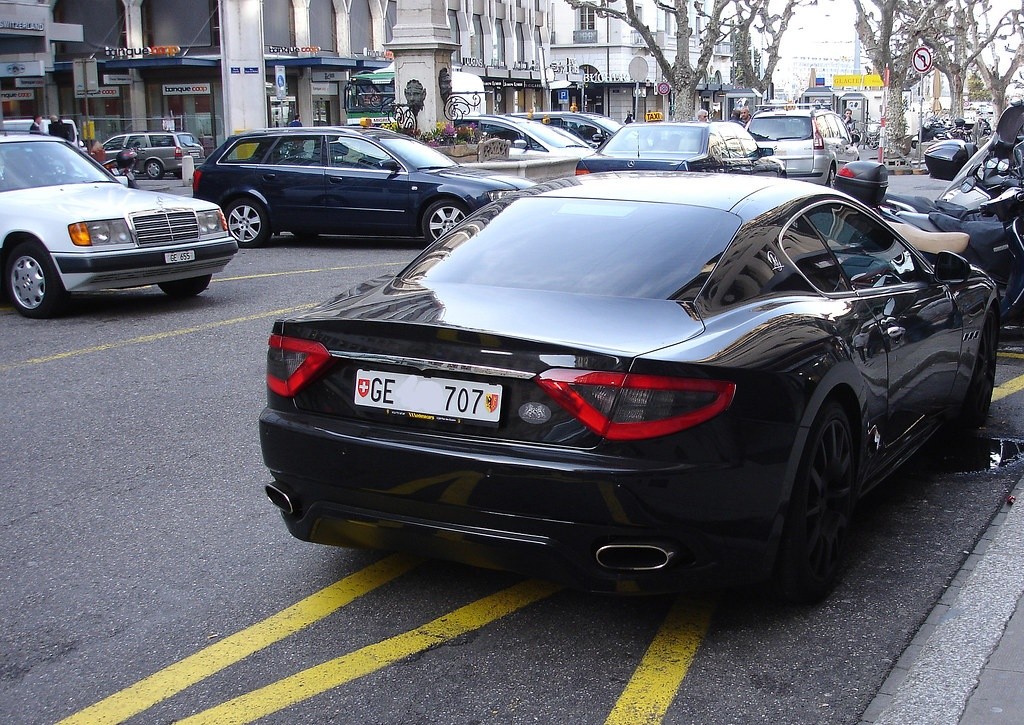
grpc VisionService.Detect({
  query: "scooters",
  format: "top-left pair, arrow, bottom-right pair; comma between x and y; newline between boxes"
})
78,140 -> 141,189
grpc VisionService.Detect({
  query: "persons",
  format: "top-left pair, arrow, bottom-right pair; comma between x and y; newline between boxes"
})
730,108 -> 752,128
698,110 -> 708,122
842,109 -> 855,139
30,114 -> 42,134
625,110 -> 633,124
439,68 -> 452,104
289,115 -> 303,144
48,116 -> 68,140
404,80 -> 426,116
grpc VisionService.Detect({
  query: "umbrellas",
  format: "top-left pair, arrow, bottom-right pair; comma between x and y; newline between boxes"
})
932,68 -> 942,113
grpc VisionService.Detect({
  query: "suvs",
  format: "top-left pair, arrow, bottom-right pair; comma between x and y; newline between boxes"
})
745,102 -> 860,190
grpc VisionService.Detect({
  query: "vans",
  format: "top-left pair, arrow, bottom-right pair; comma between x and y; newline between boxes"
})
0,118 -> 79,148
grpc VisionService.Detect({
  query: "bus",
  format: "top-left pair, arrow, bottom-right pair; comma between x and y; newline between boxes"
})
344,62 -> 487,128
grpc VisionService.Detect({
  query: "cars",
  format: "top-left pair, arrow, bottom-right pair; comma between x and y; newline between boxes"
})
451,114 -> 597,157
193,118 -> 539,249
258,172 -> 1002,608
0,134 -> 239,319
503,103 -> 625,151
576,111 -> 787,179
102,131 -> 206,181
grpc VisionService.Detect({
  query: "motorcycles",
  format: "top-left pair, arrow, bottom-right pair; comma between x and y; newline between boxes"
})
832,98 -> 1024,334
